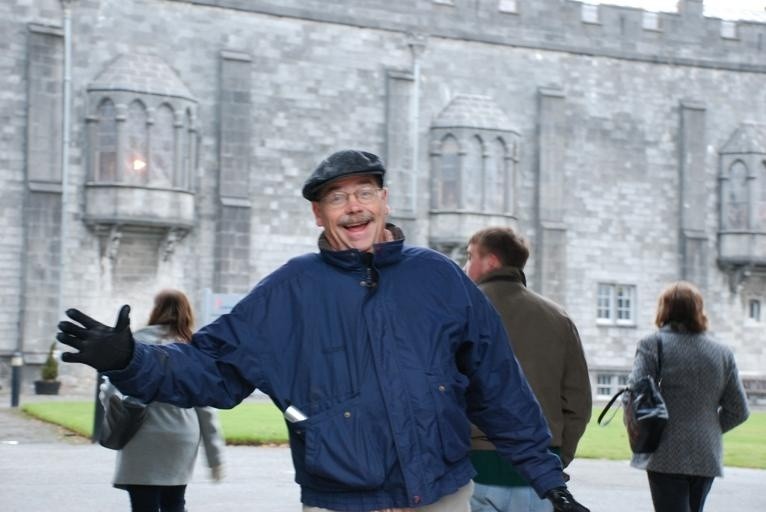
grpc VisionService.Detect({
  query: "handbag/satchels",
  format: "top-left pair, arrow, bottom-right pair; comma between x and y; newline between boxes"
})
98,390 -> 149,450
597,330 -> 670,454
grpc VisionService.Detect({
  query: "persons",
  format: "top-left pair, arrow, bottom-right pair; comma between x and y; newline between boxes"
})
460,226 -> 594,512
54,149 -> 594,512
97,288 -> 228,512
621,281 -> 752,511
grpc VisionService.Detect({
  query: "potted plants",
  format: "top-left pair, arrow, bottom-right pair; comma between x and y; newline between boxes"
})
33,342 -> 62,394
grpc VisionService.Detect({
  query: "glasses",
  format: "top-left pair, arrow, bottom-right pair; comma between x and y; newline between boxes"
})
317,188 -> 381,207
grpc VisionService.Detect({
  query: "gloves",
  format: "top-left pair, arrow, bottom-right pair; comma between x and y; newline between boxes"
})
552,490 -> 589,512
56,304 -> 134,375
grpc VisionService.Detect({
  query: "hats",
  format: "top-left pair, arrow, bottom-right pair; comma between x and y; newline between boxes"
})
301,150 -> 386,202
